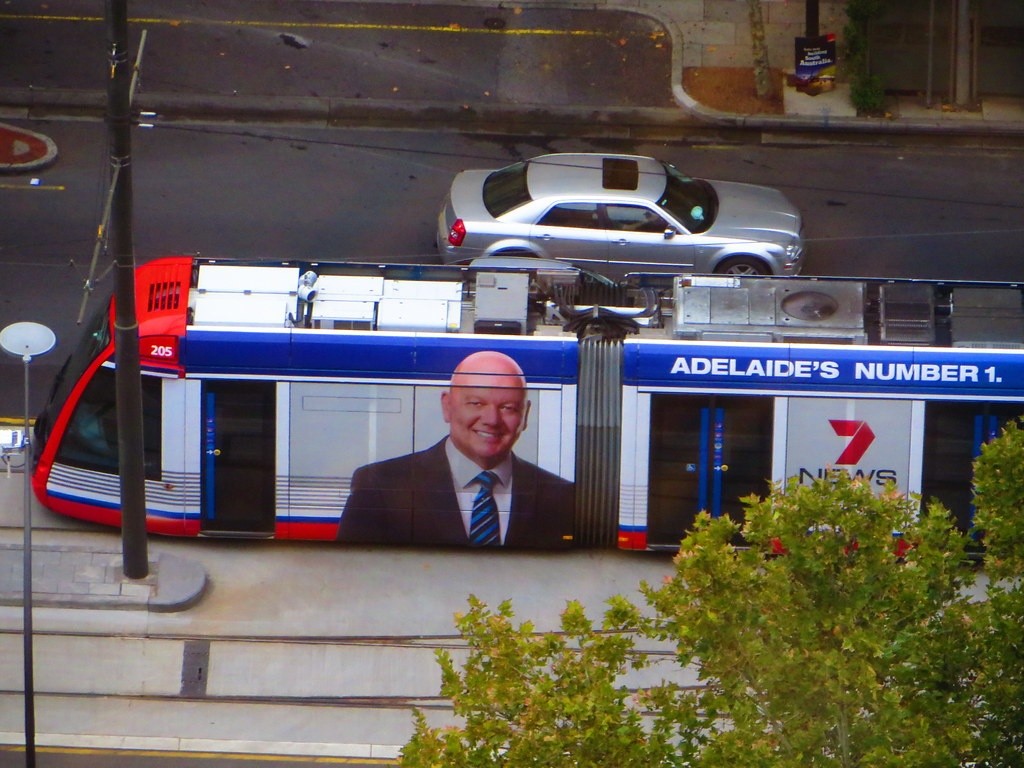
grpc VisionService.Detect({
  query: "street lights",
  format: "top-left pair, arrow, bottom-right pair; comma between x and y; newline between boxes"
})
0,322 -> 56,768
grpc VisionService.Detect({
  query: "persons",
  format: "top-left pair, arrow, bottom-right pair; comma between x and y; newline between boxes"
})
613,215 -> 659,230
339,350 -> 574,548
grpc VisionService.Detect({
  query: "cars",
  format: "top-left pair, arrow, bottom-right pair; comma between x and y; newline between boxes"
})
436,153 -> 808,288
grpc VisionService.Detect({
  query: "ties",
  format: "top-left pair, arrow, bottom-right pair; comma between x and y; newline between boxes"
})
469,471 -> 502,547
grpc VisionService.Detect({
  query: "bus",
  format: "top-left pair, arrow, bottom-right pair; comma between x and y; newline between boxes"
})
32,256 -> 1024,565
